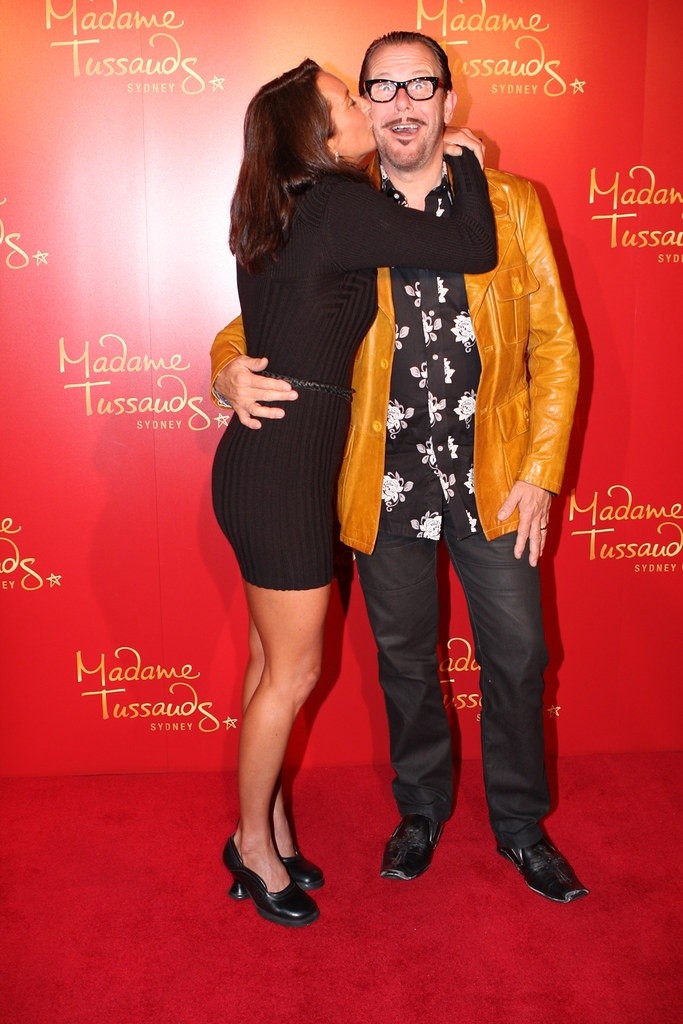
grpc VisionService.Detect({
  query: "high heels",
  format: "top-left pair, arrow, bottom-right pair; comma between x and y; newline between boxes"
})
223,832 -> 320,925
278,849 -> 325,891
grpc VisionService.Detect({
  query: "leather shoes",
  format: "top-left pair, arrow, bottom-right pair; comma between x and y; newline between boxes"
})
379,814 -> 445,880
496,835 -> 589,903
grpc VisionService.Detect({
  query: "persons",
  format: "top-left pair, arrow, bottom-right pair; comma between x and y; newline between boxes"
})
208,58 -> 500,925
210,33 -> 592,904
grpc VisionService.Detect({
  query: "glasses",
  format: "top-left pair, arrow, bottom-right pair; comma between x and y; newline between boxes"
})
365,77 -> 447,103
